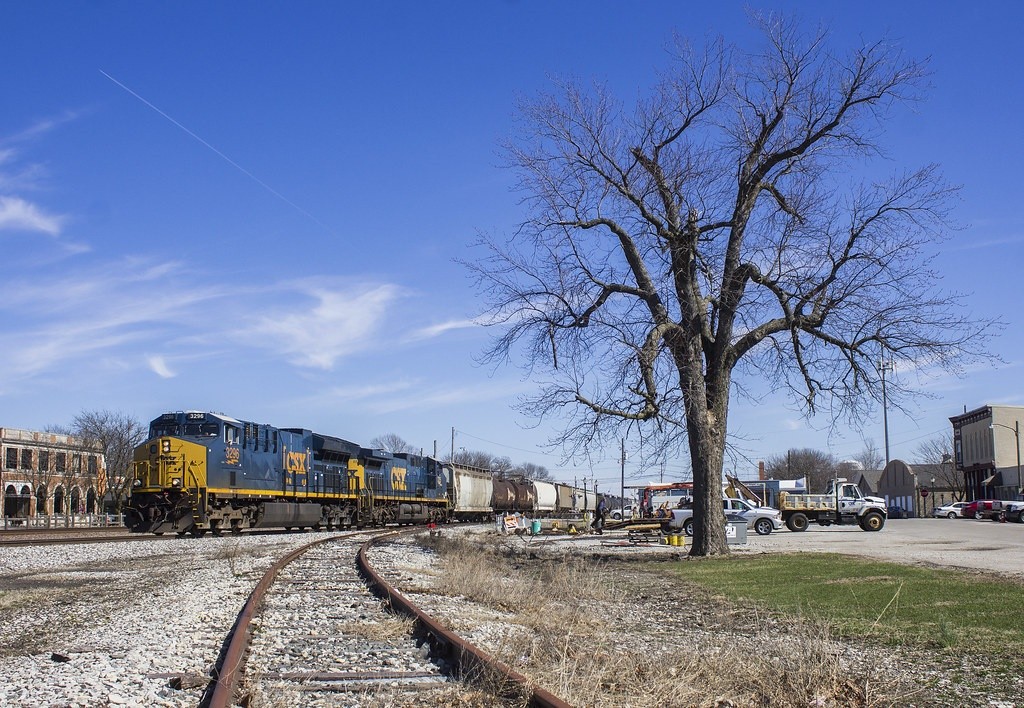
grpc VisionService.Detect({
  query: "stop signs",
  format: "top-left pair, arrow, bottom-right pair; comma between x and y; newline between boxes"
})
921,489 -> 928,497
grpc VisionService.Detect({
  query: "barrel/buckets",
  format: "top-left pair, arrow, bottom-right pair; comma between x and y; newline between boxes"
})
662,533 -> 684,546
531,520 -> 542,534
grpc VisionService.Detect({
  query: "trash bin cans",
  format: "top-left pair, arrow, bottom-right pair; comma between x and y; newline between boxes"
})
724,514 -> 748,545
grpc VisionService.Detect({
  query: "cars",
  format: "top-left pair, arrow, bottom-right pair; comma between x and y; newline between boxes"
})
930,499 -> 1024,523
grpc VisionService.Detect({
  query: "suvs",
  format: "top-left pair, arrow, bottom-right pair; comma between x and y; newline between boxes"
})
610,503 -> 640,520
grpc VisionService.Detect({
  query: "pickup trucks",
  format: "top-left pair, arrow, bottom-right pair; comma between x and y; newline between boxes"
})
670,498 -> 786,535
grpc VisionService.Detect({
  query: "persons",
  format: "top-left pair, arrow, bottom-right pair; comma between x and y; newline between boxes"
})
589,496 -> 607,527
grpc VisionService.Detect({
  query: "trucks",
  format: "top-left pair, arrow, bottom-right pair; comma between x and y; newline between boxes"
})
778,470 -> 888,531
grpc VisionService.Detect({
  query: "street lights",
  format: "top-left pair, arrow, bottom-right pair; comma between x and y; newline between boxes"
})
989,420 -> 1023,501
931,476 -> 935,509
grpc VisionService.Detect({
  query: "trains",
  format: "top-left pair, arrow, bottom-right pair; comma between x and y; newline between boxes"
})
124,409 -> 631,537
